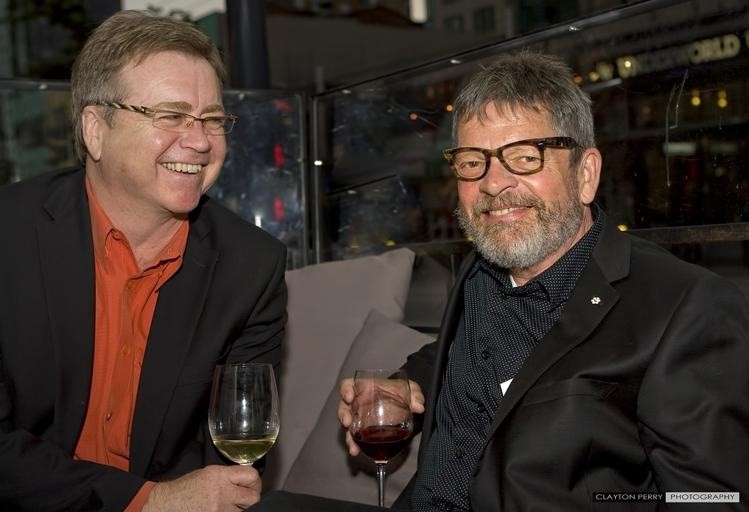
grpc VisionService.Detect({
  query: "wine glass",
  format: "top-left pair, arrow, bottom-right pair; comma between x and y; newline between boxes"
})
207,363 -> 282,468
350,370 -> 413,512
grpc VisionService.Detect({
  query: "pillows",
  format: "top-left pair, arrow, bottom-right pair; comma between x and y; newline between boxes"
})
275,246 -> 416,495
278,307 -> 437,509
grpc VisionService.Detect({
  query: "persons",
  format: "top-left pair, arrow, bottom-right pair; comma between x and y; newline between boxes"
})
1,11 -> 288,512
338,52 -> 748,511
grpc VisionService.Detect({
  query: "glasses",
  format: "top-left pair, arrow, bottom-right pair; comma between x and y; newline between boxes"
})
444,137 -> 574,182
92,101 -> 238,136
